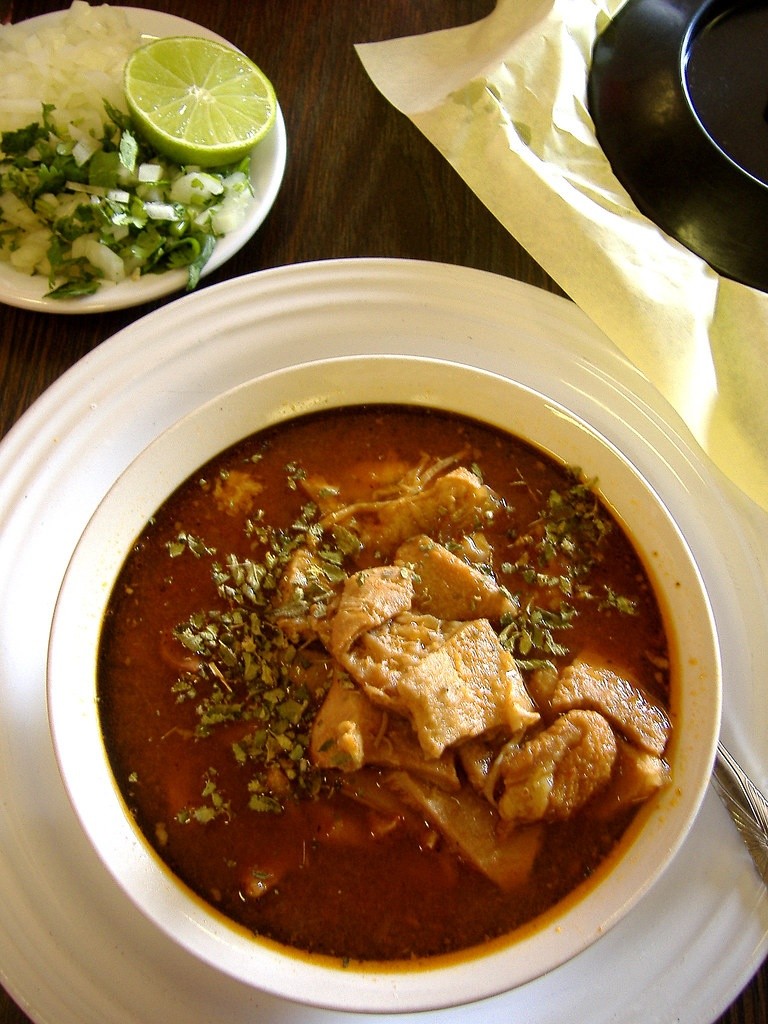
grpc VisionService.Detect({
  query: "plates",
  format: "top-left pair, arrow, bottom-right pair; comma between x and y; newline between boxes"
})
0,5 -> 288,314
0,256 -> 768,1024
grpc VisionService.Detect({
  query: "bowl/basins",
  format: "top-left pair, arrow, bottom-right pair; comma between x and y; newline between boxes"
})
584,0 -> 768,294
46,354 -> 724,1014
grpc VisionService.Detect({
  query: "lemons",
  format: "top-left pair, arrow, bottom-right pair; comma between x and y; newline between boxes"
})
122,37 -> 277,166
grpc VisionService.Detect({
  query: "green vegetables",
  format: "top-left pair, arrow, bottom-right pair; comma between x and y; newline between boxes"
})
1,98 -> 256,304
166,458 -> 636,825
